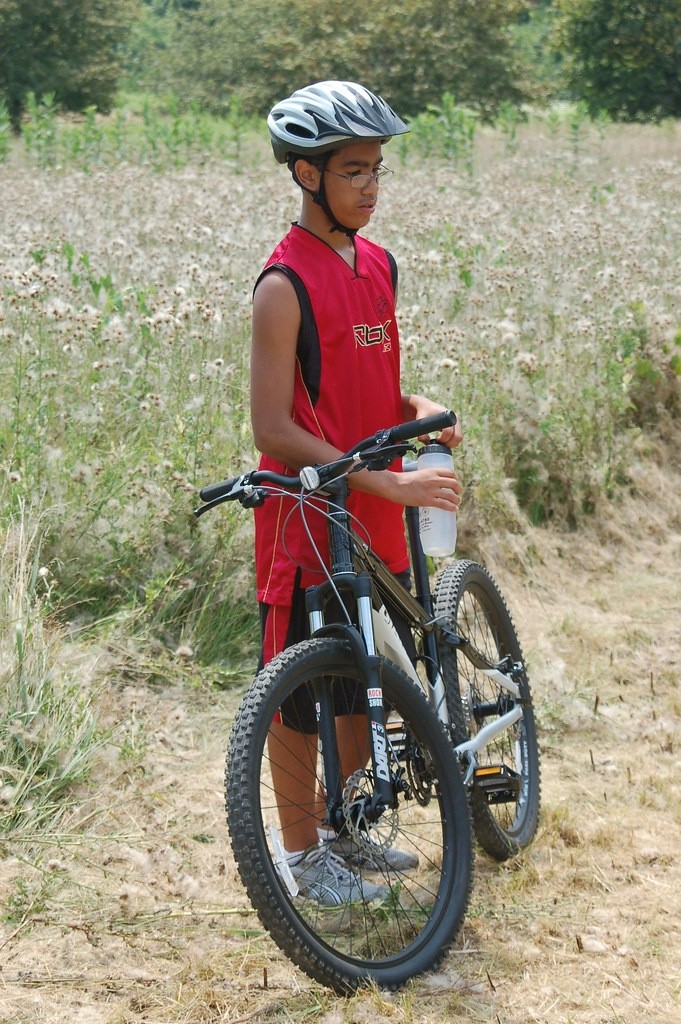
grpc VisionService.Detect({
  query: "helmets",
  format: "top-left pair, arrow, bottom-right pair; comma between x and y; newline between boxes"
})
268,81 -> 411,164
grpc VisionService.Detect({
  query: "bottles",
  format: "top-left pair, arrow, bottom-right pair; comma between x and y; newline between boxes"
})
417,438 -> 457,558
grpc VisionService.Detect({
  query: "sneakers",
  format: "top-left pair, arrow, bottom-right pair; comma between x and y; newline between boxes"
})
324,830 -> 419,876
273,838 -> 389,908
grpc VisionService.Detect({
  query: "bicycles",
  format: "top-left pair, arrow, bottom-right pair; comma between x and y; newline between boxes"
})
190,405 -> 543,1002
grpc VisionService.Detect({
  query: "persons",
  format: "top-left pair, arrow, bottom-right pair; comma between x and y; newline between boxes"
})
249,79 -> 462,910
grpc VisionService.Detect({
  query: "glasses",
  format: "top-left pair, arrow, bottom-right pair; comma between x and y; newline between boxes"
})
314,162 -> 394,189
